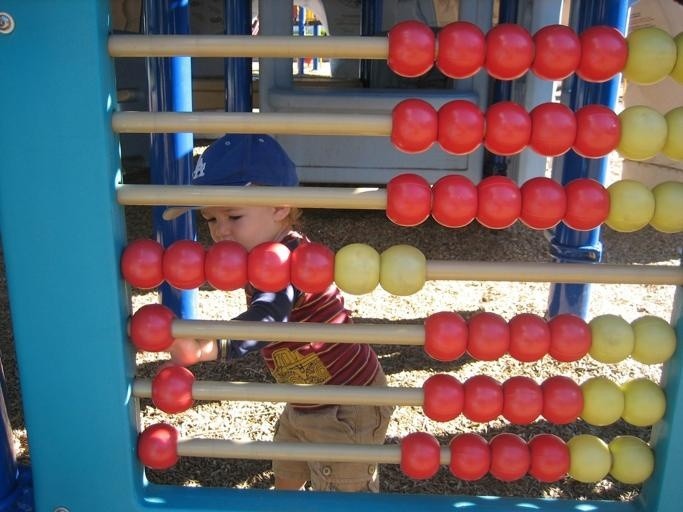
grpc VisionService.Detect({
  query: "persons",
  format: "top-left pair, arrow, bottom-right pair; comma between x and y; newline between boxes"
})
162,134 -> 398,493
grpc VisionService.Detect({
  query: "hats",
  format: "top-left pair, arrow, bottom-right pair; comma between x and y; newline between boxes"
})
162,133 -> 299,222
